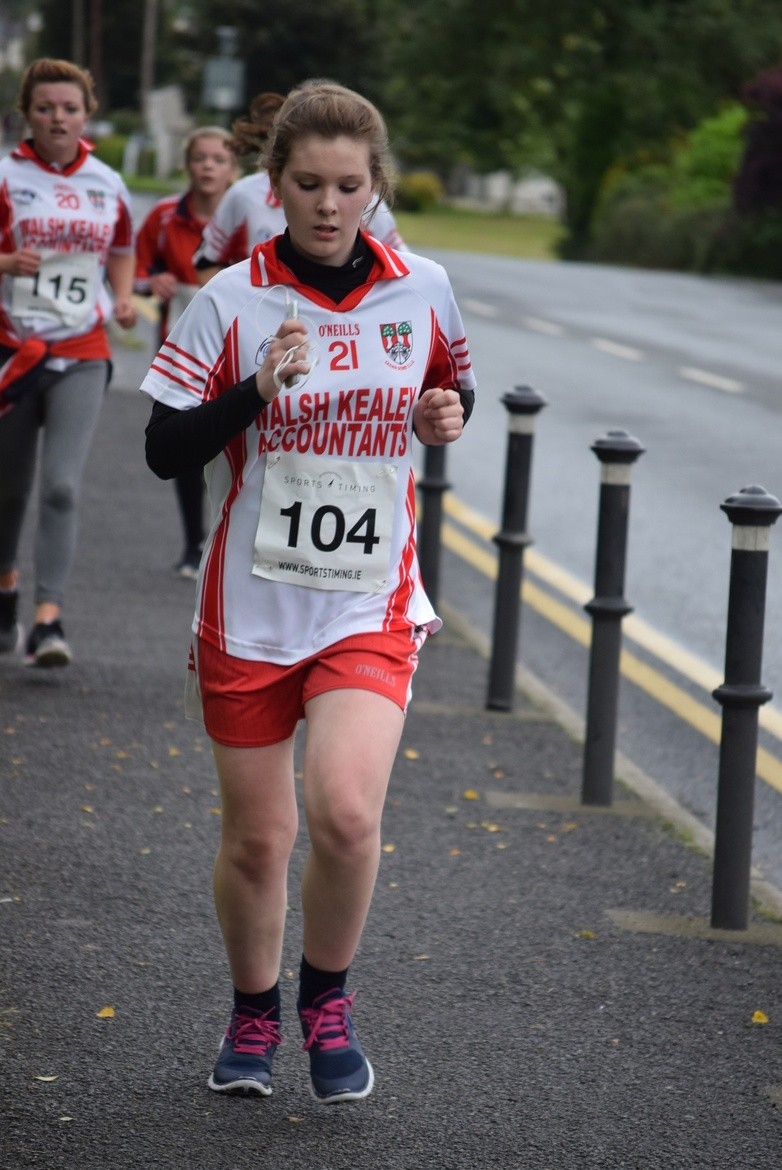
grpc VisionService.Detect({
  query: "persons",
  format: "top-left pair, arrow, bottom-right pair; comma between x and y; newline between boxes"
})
133,129 -> 244,579
0,59 -> 139,666
141,83 -> 476,1102
190,93 -> 409,286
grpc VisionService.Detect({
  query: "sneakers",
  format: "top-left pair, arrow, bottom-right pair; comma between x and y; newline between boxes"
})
208,1005 -> 283,1095
297,988 -> 375,1103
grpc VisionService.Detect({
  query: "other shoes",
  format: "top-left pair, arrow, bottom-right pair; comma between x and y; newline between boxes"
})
25,617 -> 71,666
0,588 -> 19,654
173,540 -> 206,581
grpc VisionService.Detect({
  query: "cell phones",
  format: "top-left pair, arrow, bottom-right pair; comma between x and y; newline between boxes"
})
282,297 -> 300,387
